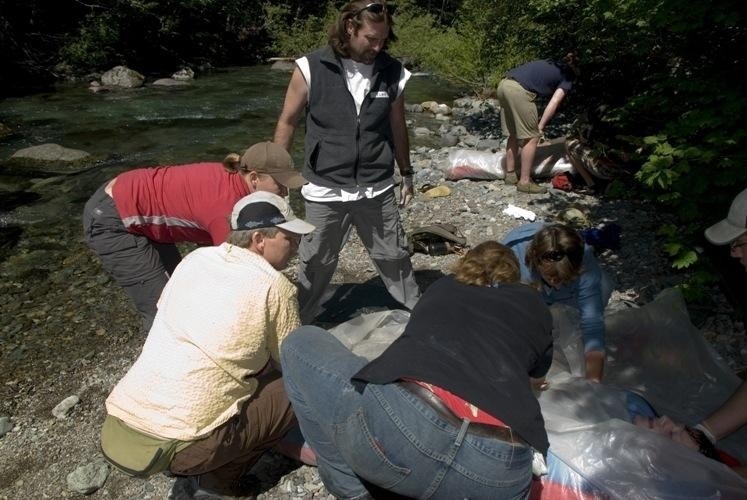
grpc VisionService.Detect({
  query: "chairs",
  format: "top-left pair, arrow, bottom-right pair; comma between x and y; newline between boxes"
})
505,168 -> 520,184
517,182 -> 547,194
198,463 -> 263,497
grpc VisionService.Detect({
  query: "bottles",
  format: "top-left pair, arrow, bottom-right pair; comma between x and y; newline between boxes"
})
240,142 -> 309,189
231,190 -> 316,235
705,189 -> 746,245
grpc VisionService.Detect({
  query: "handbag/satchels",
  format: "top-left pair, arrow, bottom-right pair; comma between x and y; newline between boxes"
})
538,128 -> 544,131
693,423 -> 717,445
400,167 -> 415,176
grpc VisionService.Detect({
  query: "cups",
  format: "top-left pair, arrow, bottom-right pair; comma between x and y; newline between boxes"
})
350,4 -> 390,18
731,240 -> 747,252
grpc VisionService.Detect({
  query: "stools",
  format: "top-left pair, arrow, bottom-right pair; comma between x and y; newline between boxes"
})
400,379 -> 527,445
542,246 -> 579,262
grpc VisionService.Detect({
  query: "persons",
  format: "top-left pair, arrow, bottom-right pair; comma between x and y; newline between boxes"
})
272,0 -> 424,326
279,240 -> 554,499
624,388 -> 721,464
688,186 -> 747,453
82,140 -> 309,337
500,221 -> 614,384
496,52 -> 579,194
565,108 -> 630,194
99,190 -> 316,500
